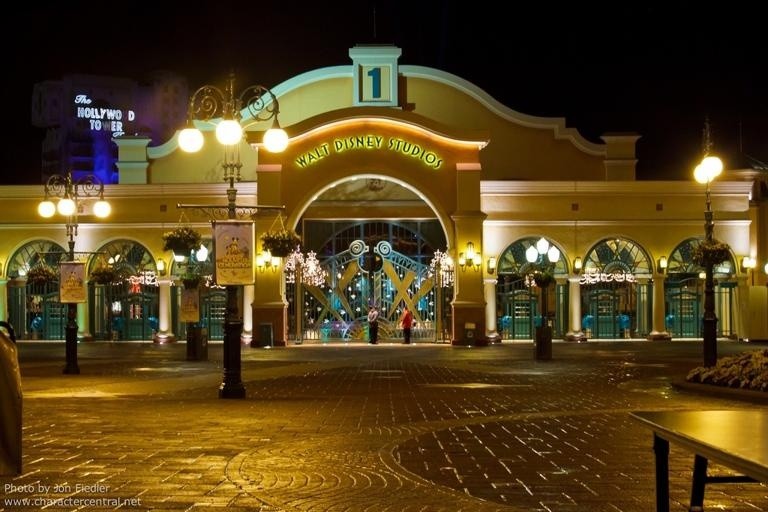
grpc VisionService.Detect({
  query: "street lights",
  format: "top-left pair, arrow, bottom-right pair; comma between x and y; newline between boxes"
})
693,114 -> 724,367
526,237 -> 560,361
37,164 -> 112,374
173,244 -> 209,361
178,65 -> 289,398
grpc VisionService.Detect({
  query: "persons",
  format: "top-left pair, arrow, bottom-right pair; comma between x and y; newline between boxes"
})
400,306 -> 412,344
367,306 -> 378,344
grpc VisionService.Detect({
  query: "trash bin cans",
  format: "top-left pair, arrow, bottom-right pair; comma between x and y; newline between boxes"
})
533,326 -> 552,360
185,327 -> 208,362
257,323 -> 273,347
464,328 -> 481,345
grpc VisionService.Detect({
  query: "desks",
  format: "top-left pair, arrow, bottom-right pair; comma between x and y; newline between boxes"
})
627,410 -> 768,512
